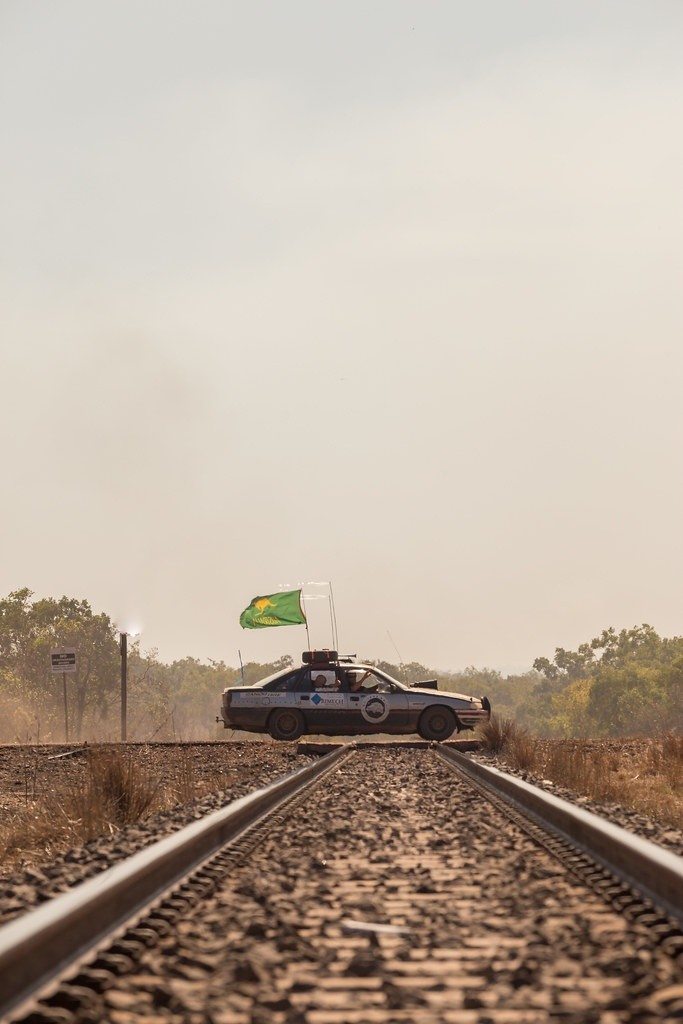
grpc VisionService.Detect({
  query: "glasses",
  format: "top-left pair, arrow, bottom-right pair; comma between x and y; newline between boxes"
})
349,676 -> 357,679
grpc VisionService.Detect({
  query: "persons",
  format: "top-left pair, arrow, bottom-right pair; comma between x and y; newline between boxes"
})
311,675 -> 342,692
346,669 -> 380,692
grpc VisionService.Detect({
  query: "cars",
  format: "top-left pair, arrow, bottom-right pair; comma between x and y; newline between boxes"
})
214,647 -> 492,741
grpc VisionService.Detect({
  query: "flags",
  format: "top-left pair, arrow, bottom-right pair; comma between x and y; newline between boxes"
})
240,589 -> 306,629
